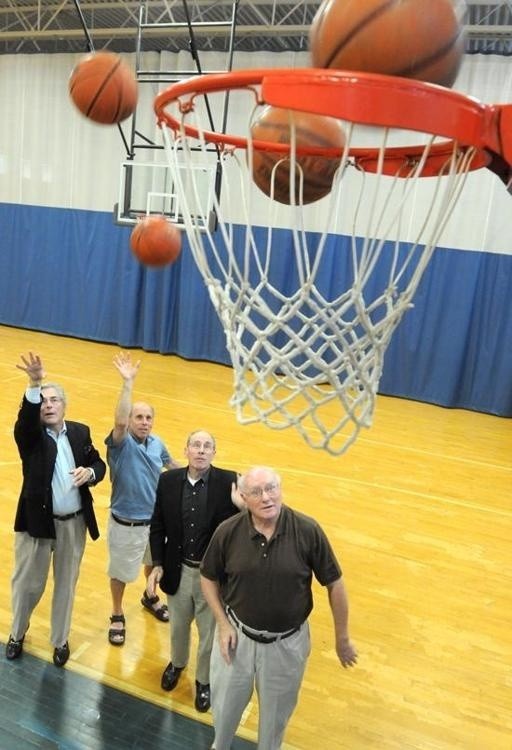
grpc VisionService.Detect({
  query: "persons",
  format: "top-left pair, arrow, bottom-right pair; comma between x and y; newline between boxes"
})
6,351 -> 106,666
103,350 -> 182,646
147,429 -> 247,712
199,466 -> 360,750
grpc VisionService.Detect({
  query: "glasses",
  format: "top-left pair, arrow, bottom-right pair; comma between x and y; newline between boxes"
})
239,483 -> 279,496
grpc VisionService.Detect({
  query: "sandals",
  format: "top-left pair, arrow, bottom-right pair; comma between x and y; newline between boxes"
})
108,615 -> 126,645
142,591 -> 170,622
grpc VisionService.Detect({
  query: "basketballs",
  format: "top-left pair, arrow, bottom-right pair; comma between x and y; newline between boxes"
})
246,106 -> 347,206
128,213 -> 183,269
309,0 -> 467,91
65,49 -> 139,126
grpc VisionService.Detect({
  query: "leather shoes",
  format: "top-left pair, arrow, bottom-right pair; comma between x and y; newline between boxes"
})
53,642 -> 70,665
6,637 -> 26,659
195,681 -> 211,711
160,663 -> 186,691
